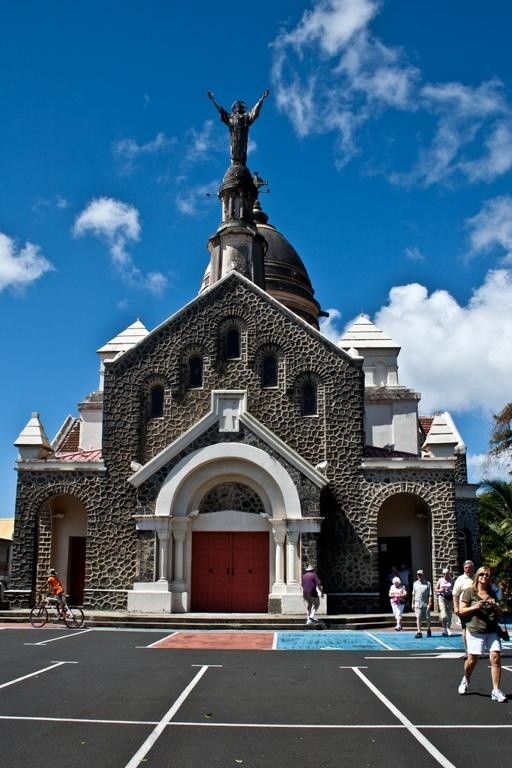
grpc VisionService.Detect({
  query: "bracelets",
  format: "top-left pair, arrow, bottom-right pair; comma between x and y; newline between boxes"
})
492,601 -> 497,608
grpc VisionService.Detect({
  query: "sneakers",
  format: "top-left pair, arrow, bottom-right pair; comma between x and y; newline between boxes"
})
395,625 -> 403,631
306,614 -> 320,625
426,629 -> 431,637
415,633 -> 422,638
442,628 -> 452,636
458,680 -> 469,695
491,689 -> 507,703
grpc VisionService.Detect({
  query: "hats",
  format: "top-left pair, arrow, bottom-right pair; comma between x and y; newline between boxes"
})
417,570 -> 424,575
304,565 -> 316,571
48,569 -> 59,577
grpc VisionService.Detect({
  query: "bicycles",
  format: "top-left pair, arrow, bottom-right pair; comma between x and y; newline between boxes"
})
29,591 -> 84,629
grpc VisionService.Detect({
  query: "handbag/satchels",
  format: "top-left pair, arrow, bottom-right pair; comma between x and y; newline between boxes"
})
443,591 -> 453,601
497,626 -> 510,641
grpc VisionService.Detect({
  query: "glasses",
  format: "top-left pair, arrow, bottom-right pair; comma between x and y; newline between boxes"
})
478,573 -> 489,577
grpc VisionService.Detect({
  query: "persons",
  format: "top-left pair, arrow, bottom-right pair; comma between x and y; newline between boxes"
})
450,559 -> 481,660
434,568 -> 456,637
455,566 -> 508,702
390,561 -> 410,613
410,570 -> 433,638
38,567 -> 75,620
301,565 -> 324,625
388,576 -> 407,630
206,88 -> 270,165
